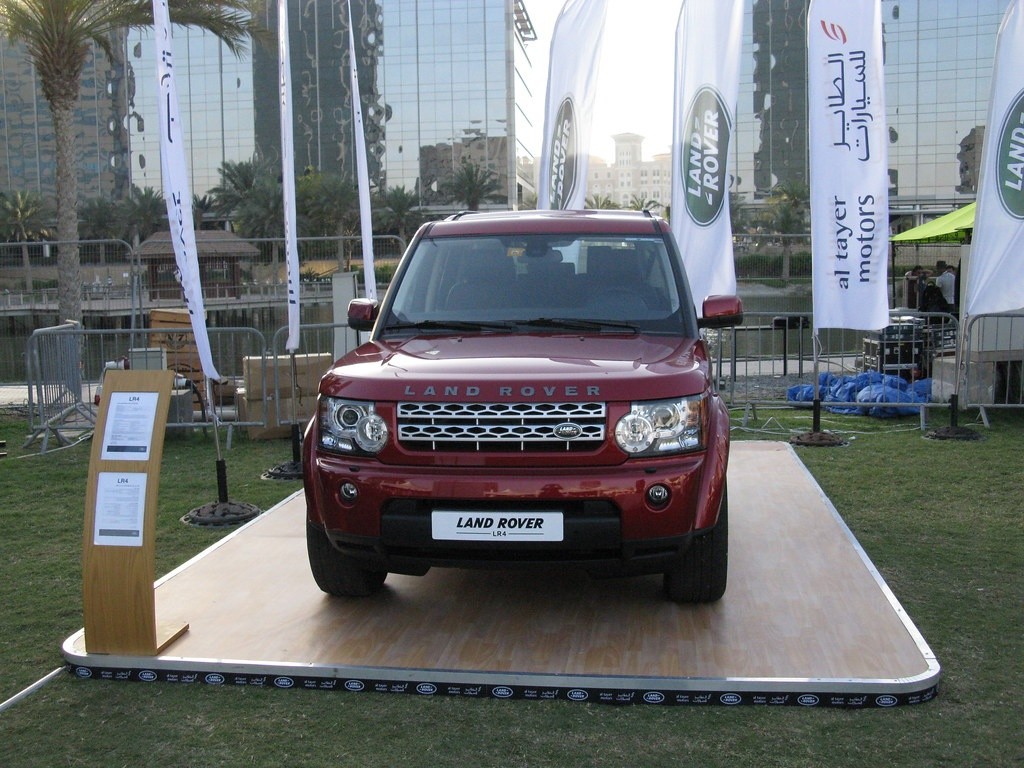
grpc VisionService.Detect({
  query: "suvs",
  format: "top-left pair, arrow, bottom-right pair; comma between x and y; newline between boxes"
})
301,206 -> 744,604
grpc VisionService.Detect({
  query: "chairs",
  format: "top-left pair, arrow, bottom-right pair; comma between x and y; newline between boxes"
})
441,240 -> 527,310
521,261 -> 583,312
586,249 -> 673,318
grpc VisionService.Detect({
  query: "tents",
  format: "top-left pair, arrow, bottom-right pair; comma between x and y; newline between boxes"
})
889,201 -> 976,309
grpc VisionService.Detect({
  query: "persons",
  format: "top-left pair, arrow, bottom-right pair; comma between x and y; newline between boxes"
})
946,265 -> 954,274
936,260 -> 955,305
905,265 -> 933,309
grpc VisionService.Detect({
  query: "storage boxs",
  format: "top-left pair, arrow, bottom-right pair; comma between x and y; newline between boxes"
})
148,307 -> 333,441
861,318 -> 956,384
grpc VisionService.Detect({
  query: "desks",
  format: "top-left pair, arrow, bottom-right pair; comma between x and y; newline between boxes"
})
721,326 -> 810,382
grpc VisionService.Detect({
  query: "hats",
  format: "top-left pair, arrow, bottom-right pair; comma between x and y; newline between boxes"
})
936,261 -> 946,267
